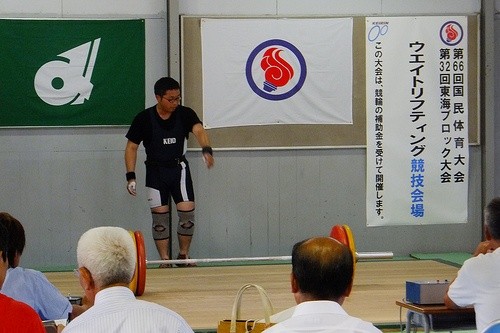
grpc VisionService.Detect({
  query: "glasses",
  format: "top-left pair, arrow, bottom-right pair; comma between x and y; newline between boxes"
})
73,267 -> 82,275
158,95 -> 182,103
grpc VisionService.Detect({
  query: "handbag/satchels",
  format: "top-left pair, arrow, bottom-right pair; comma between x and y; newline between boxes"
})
216,283 -> 278,333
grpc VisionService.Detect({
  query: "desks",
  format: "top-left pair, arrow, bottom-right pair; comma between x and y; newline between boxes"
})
395,300 -> 477,333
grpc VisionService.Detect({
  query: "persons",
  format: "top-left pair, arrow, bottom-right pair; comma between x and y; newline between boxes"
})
61,227 -> 195,333
125,76 -> 214,267
0,212 -> 92,333
443,197 -> 500,333
260,235 -> 384,333
